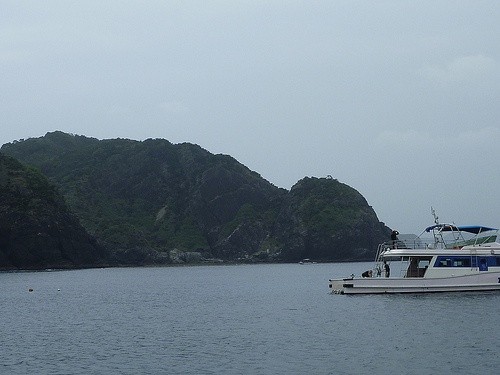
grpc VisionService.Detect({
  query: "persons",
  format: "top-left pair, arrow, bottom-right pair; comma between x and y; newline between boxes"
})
391,231 -> 397,249
383,261 -> 390,277
362,271 -> 369,277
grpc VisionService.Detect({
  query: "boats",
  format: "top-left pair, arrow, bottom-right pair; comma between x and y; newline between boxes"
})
328,204 -> 500,296
299,258 -> 318,265
